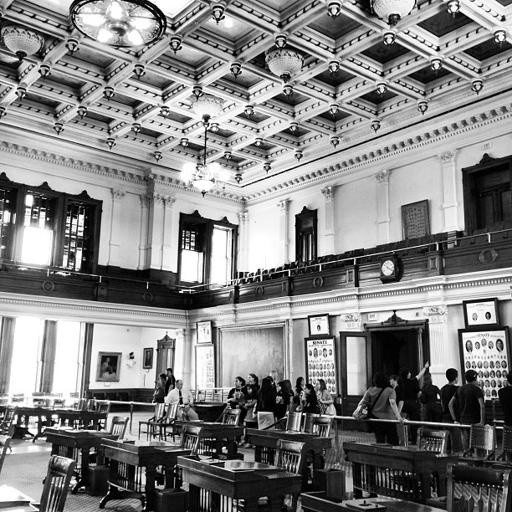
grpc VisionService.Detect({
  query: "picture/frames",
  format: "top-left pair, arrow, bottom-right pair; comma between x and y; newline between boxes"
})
307,313 -> 330,336
194,344 -> 217,393
457,325 -> 510,400
197,319 -> 213,344
399,198 -> 431,239
143,347 -> 154,369
304,335 -> 339,397
95,349 -> 123,383
462,297 -> 499,326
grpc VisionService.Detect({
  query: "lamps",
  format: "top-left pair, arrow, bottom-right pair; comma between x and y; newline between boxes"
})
260,35 -> 304,82
190,89 -> 226,127
368,0 -> 418,29
67,0 -> 165,49
176,117 -> 228,196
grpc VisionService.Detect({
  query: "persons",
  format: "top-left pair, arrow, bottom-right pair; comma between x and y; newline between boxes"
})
101,357 -> 116,378
317,324 -> 320,331
353,361 -> 512,450
306,348 -> 335,395
472,312 -> 479,321
227,373 -> 338,424
154,368 -> 199,421
465,339 -> 507,398
485,312 -> 492,321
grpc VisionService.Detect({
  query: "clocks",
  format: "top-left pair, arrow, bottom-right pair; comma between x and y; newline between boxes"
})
378,253 -> 403,284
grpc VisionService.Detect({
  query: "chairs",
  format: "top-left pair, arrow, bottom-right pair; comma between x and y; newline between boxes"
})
227,221 -> 512,284
0,390 -> 264,511
264,404 -> 512,512
